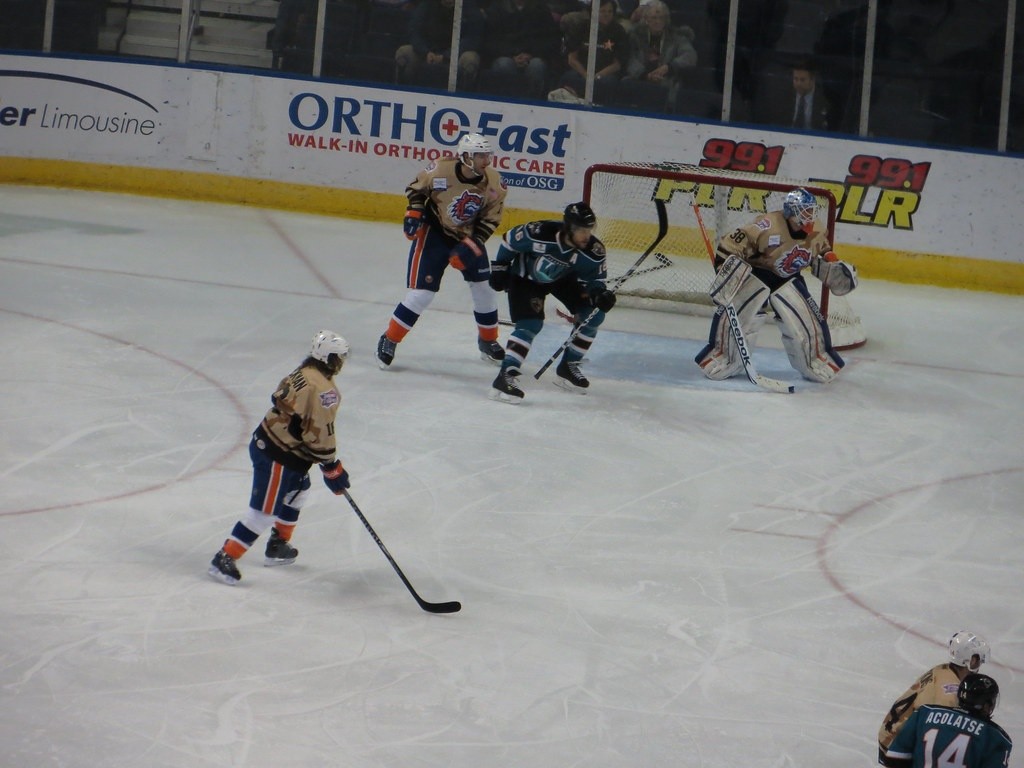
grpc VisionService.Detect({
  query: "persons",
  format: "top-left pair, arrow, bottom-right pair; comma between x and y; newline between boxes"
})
694,189 -> 858,382
393,0 -> 698,110
375,134 -> 505,370
207,330 -> 352,585
884,673 -> 1012,768
781,63 -> 831,130
877,630 -> 991,768
489,201 -> 617,405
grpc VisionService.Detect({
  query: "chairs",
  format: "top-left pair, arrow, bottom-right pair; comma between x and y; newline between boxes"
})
575,0 -> 1024,150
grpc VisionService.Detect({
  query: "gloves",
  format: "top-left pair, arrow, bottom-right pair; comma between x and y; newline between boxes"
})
319,459 -> 350,495
447,237 -> 483,272
489,260 -> 512,293
403,206 -> 424,239
594,289 -> 616,313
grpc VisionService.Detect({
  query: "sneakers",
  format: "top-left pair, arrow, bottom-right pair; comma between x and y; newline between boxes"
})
489,366 -> 525,398
375,332 -> 396,365
478,337 -> 505,359
265,528 -> 298,559
211,552 -> 241,580
553,347 -> 590,387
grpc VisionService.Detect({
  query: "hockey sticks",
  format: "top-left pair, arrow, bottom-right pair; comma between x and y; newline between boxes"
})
606,251 -> 674,284
688,191 -> 795,395
342,489 -> 462,614
533,197 -> 669,381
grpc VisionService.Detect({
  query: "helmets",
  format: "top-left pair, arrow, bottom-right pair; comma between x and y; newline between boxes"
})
784,187 -> 822,223
958,674 -> 1000,713
565,202 -> 596,228
458,134 -> 494,157
948,630 -> 992,665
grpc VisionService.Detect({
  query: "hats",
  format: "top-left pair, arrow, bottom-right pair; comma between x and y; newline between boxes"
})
308,329 -> 351,364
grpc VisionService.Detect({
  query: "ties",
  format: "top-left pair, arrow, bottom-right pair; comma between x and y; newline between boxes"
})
794,95 -> 806,132
